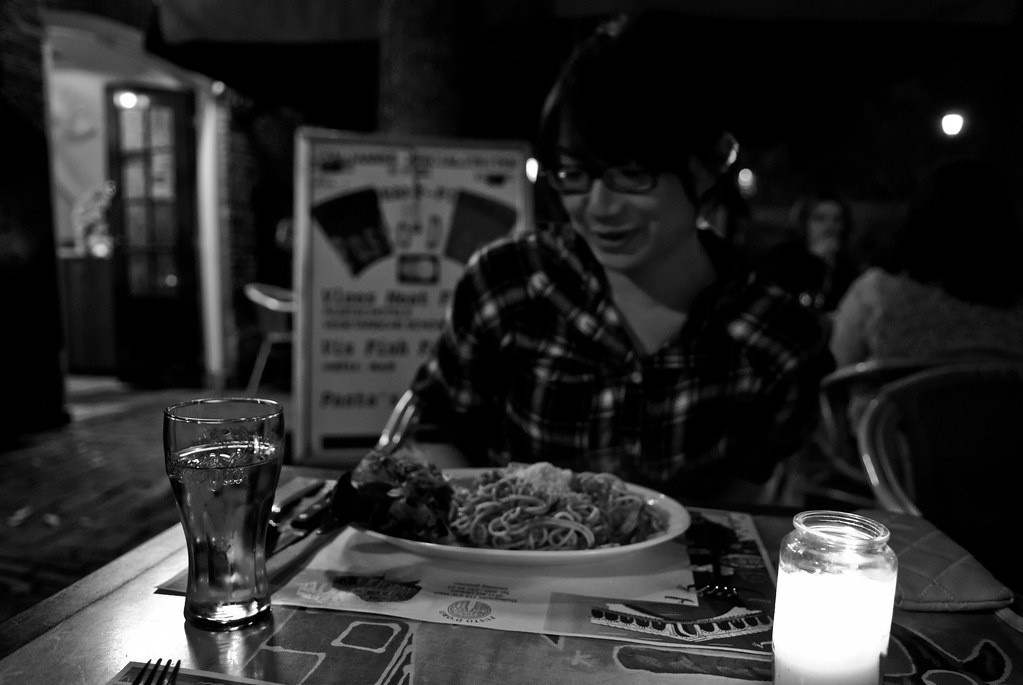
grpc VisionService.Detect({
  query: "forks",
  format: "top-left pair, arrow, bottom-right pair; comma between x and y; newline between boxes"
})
132,658 -> 181,685
695,549 -> 737,599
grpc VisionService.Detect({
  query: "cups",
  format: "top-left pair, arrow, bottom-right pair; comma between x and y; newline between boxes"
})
163,398 -> 285,632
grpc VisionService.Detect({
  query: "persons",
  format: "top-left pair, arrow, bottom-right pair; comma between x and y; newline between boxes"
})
396,13 -> 822,507
769,193 -> 864,311
826,162 -> 1023,366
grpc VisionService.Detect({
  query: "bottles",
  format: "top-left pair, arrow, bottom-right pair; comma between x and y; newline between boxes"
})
772,510 -> 898,685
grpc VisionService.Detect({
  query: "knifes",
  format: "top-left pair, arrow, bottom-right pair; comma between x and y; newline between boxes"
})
271,486 -> 336,553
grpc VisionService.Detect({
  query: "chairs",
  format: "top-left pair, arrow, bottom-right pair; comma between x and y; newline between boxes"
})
245,284 -> 292,392
807,349 -> 1023,643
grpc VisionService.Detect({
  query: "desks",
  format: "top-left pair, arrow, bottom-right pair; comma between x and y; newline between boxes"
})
0,477 -> 1023,685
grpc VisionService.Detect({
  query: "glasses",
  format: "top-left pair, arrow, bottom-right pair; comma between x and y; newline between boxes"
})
538,161 -> 672,194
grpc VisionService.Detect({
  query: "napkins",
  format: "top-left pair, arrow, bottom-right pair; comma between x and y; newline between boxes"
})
858,508 -> 1015,612
156,480 -> 349,594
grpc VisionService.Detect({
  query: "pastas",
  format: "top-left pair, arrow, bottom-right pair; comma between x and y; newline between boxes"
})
450,466 -> 668,550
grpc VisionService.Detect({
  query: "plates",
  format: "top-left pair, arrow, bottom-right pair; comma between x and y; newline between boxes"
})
352,467 -> 691,565
